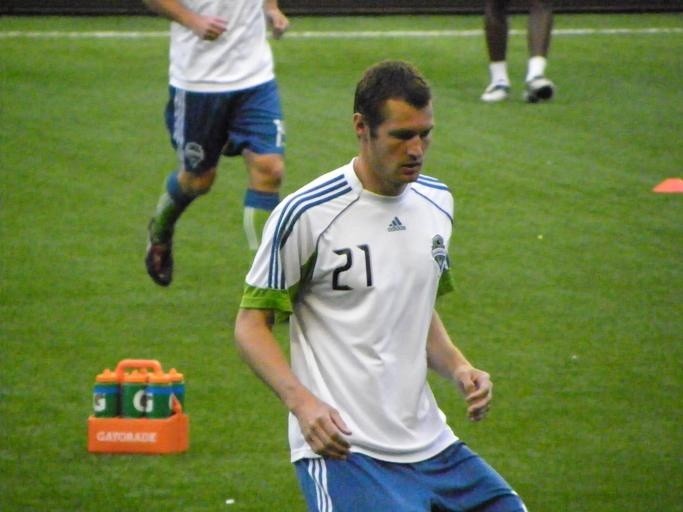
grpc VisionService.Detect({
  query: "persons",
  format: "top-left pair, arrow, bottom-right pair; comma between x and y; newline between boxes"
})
479,0 -> 556,103
234,59 -> 526,512
145,0 -> 290,287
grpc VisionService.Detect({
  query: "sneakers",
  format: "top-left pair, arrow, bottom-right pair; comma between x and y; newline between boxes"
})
145,217 -> 174,286
523,76 -> 555,103
481,77 -> 510,103
